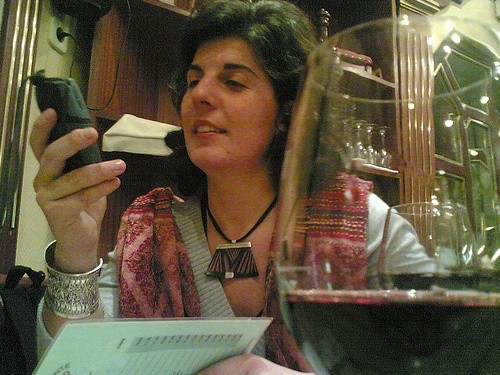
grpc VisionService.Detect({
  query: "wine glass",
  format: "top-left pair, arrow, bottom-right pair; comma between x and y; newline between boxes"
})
339,112 -> 394,169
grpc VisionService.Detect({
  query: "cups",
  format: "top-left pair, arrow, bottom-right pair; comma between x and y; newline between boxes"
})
378,199 -> 482,291
273,14 -> 500,375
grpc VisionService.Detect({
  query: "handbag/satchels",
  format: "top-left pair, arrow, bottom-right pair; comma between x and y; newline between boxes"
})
0,265 -> 47,375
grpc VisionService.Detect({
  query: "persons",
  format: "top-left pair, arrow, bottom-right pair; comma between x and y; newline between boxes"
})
28,1 -> 434,375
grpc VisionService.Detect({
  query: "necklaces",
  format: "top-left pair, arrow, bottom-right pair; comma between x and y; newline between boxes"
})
203,193 -> 278,278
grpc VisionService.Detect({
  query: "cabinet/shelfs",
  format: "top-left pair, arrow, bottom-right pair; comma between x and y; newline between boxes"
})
88,1 -> 500,266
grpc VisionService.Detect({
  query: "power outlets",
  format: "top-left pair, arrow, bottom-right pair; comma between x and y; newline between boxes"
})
47,17 -> 68,54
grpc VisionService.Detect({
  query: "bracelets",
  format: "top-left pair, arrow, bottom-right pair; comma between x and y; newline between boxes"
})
43,239 -> 105,319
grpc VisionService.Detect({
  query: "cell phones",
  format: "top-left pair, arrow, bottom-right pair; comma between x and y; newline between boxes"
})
34,78 -> 102,172
